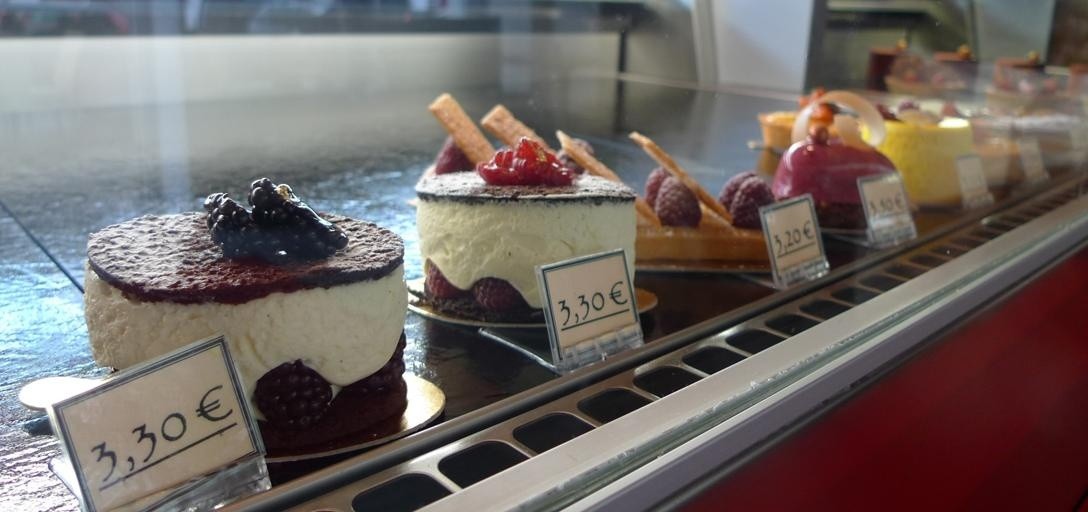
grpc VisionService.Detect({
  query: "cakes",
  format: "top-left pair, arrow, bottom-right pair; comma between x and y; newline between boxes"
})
761,42 -> 1087,228
83,177 -> 407,452
414,139 -> 638,319
412,90 -> 777,264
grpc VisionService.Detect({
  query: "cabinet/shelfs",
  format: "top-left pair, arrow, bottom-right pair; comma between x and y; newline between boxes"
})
1,0 -> 1087,512
